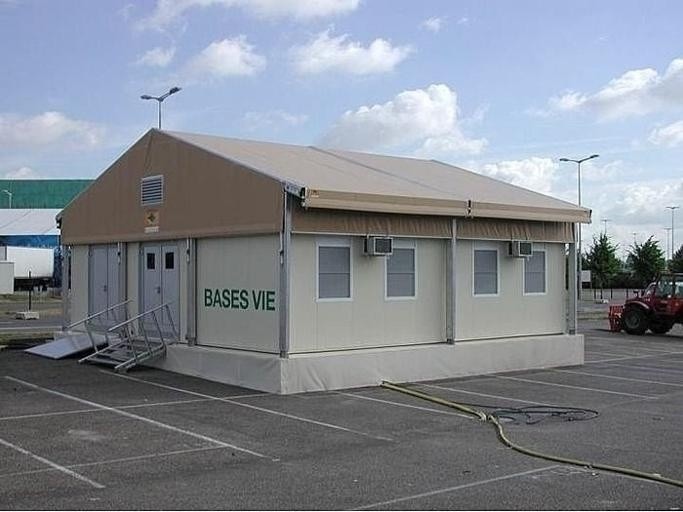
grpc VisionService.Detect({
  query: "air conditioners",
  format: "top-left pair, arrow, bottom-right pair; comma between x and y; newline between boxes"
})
362,234 -> 393,257
506,241 -> 533,258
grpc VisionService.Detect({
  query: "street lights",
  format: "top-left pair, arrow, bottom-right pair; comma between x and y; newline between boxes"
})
135,82 -> 182,128
600,216 -> 611,236
2,188 -> 12,208
662,203 -> 678,259
557,148 -> 601,300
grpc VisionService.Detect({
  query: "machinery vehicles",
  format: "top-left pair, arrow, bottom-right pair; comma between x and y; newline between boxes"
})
608,272 -> 683,334
